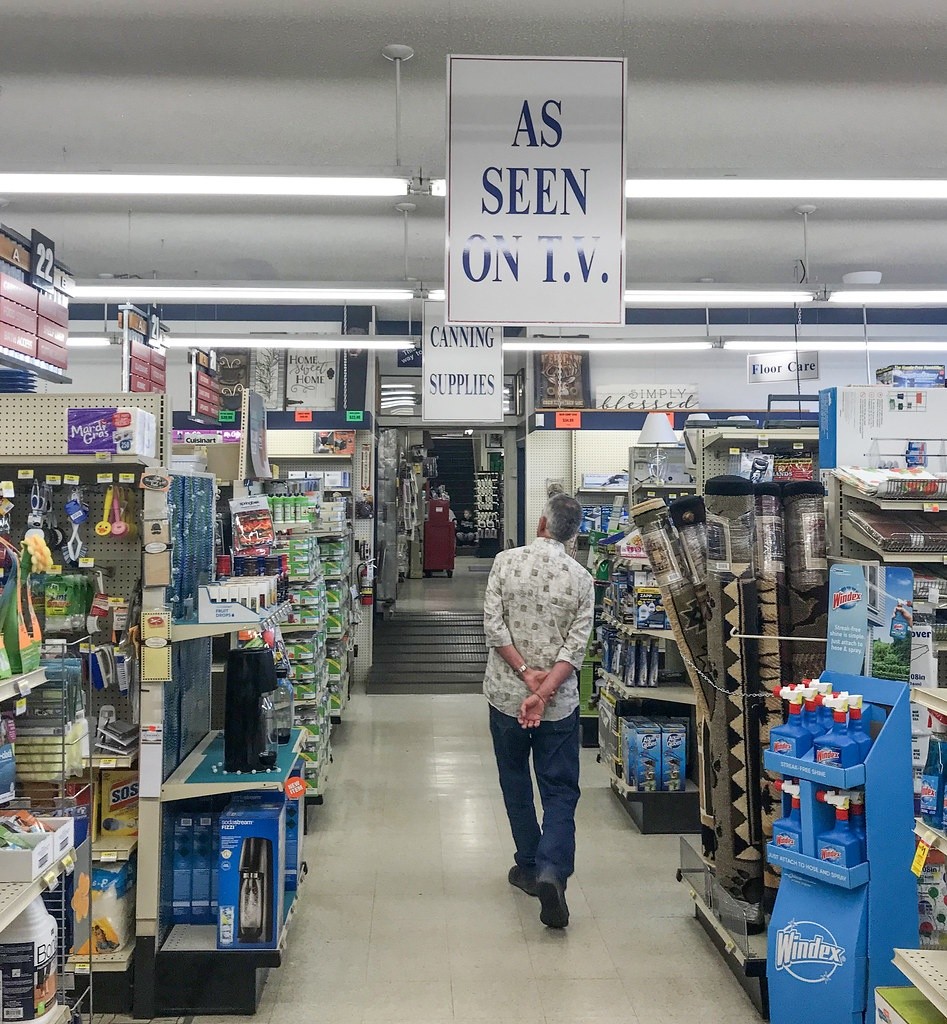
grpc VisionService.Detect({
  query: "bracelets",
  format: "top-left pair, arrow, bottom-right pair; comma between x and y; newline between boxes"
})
534,691 -> 547,705
514,661 -> 528,678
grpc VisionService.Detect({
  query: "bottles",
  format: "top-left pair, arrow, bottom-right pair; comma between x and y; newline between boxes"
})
255,667 -> 295,768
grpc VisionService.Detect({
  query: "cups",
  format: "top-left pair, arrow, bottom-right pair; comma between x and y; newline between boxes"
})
241,872 -> 262,937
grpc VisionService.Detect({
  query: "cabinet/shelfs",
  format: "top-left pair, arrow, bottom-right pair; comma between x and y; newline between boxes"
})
0,387 -> 946,1024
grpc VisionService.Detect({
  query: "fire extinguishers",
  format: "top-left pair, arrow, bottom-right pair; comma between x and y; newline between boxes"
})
353,558 -> 378,605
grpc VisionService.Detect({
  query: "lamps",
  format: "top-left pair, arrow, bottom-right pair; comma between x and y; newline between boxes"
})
426,288 -> 947,308
503,337 -> 717,351
160,335 -> 417,350
0,173 -> 411,196
723,336 -> 947,352
66,280 -> 417,303
431,178 -> 946,201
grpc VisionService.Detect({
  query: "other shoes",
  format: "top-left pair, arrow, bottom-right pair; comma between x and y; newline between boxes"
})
508,864 -> 569,928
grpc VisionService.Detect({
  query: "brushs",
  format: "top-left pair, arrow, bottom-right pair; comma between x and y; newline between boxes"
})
20,478 -> 67,552
88,642 -> 129,698
61,486 -> 88,564
94,483 -> 138,539
86,569 -> 106,635
0,497 -> 13,568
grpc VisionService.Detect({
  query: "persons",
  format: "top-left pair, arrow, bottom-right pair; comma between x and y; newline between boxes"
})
481,491 -> 597,932
